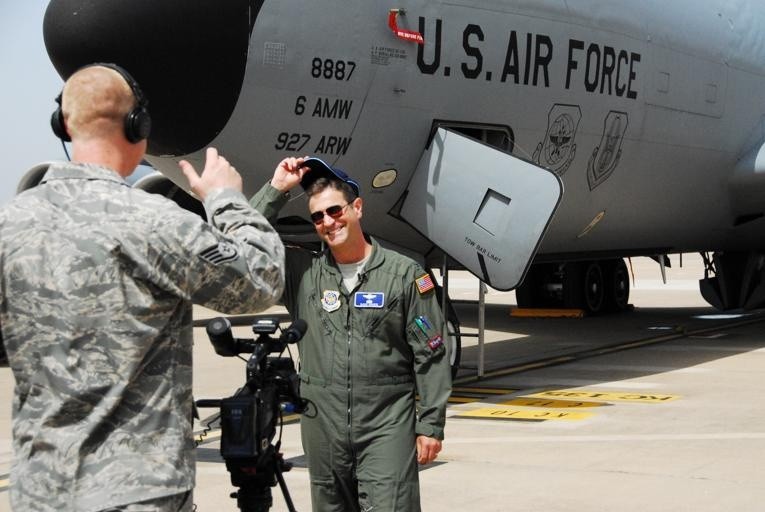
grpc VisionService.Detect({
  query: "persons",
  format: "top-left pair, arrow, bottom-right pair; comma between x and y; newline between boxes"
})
1,63 -> 287,512
246,154 -> 453,512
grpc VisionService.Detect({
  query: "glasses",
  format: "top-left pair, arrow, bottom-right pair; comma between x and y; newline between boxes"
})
308,199 -> 355,225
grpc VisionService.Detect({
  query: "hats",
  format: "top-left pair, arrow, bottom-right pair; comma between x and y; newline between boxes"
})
297,158 -> 360,197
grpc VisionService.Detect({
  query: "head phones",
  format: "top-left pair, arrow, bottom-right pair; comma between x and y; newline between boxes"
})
52,62 -> 151,144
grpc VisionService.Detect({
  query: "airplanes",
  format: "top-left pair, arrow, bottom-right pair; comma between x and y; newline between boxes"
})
16,0 -> 765,389
